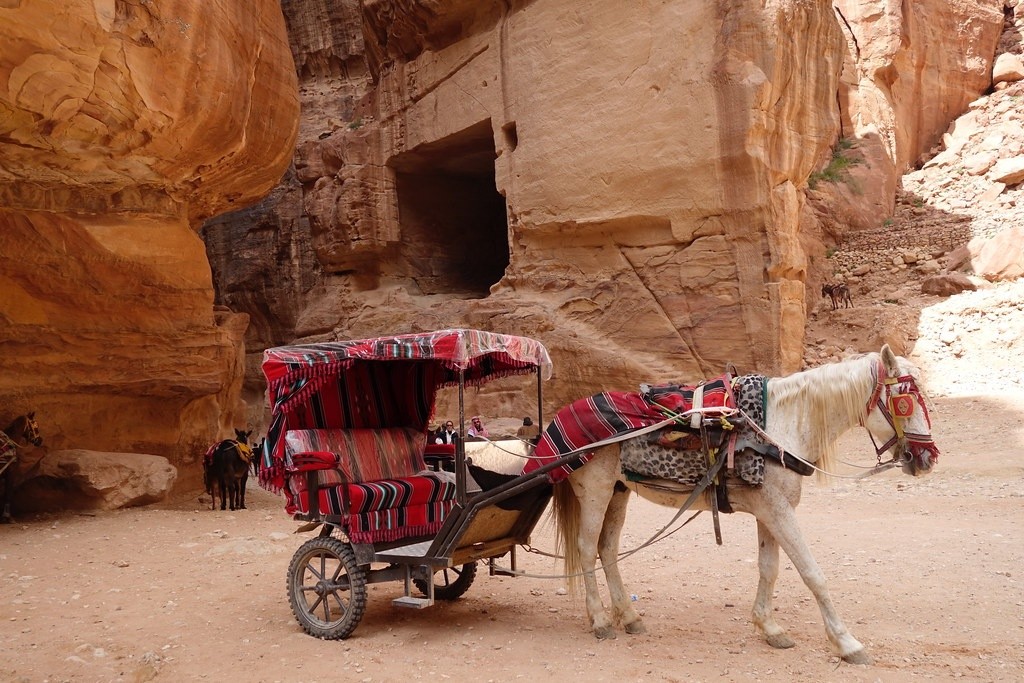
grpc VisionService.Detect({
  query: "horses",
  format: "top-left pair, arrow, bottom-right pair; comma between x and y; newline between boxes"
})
0,411 -> 43,525
202,428 -> 252,512
536,342 -> 941,666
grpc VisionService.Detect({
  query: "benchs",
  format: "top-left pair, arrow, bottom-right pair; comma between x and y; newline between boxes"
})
284,425 -> 461,542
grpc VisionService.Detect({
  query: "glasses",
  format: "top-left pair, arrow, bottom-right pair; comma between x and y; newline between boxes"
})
448,424 -> 452,426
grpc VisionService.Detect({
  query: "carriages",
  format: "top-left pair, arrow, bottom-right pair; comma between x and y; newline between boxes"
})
255,327 -> 938,665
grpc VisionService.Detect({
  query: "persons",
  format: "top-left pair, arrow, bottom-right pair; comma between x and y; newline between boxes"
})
438,421 -> 459,473
252,443 -> 260,476
516,417 -> 539,437
468,417 -> 490,437
